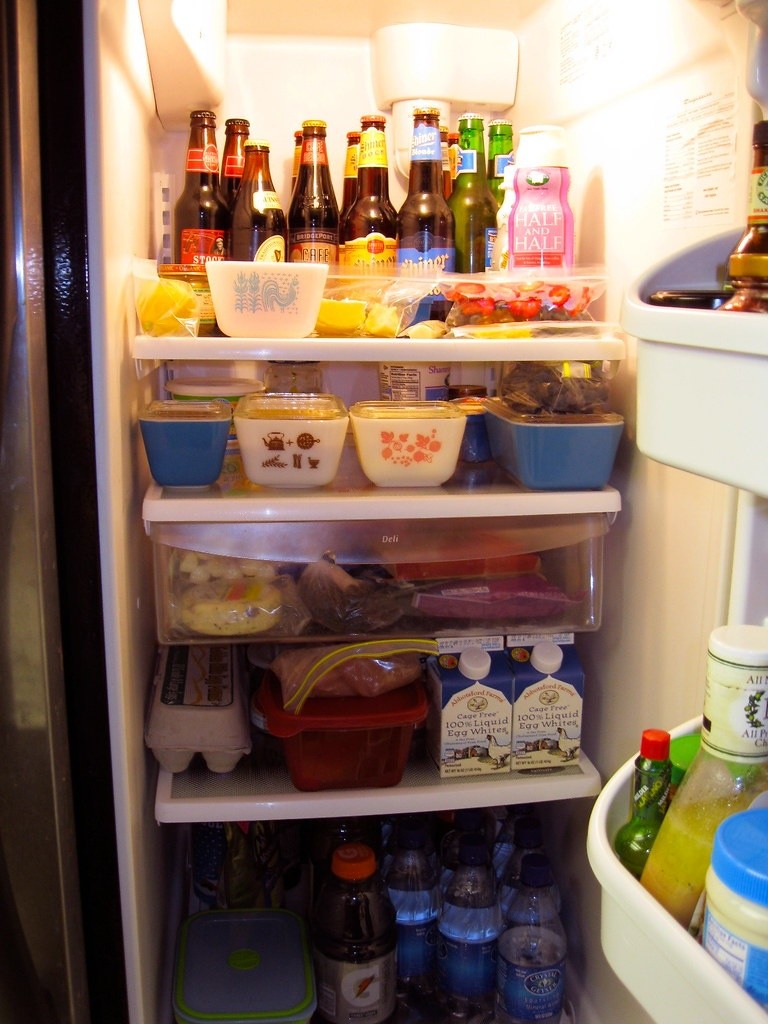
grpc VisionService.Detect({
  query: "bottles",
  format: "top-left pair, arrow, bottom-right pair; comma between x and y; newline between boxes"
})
168,377 -> 266,456
339,132 -> 361,274
715,253 -> 768,314
291,131 -> 303,193
722,120 -> 768,290
702,808 -> 768,1011
174,111 -> 231,265
288,120 -> 340,274
440,113 -> 574,278
231,140 -> 288,262
641,624 -> 768,947
615,728 -> 674,882
379,362 -> 451,401
346,114 -> 398,275
312,803 -> 567,1024
398,108 -> 455,326
265,360 -> 322,392
220,119 -> 250,206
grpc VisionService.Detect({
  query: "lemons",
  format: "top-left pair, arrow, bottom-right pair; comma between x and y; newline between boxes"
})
316,298 -> 402,338
138,279 -> 199,337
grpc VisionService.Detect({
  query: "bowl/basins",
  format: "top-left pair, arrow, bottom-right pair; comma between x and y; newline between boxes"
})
140,399 -> 230,489
234,394 -> 349,488
204,262 -> 329,338
485,399 -> 624,490
349,401 -> 467,487
258,680 -> 428,791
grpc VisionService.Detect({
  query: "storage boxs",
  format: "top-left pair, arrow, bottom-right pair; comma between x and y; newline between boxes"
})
168,906 -> 318,1024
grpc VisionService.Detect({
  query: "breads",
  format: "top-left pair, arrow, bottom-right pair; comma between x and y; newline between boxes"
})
173,577 -> 284,635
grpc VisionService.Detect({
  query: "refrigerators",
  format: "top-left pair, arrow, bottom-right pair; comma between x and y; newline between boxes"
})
0,0 -> 767,1024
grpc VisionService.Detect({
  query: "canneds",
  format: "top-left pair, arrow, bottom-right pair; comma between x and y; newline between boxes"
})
156,263 -> 218,337
701,806 -> 768,1016
